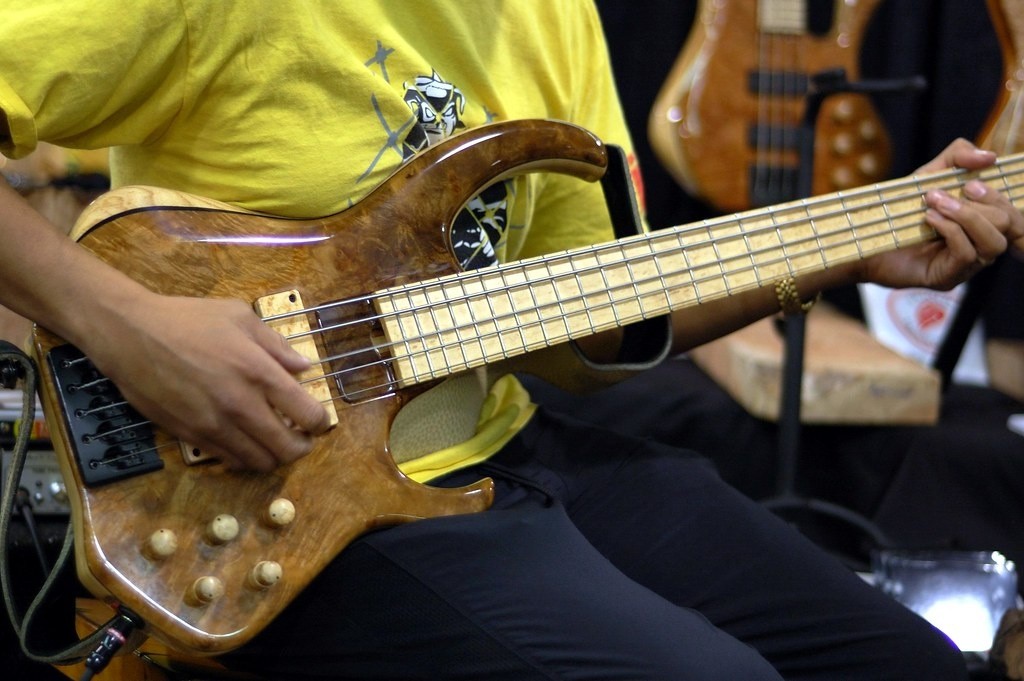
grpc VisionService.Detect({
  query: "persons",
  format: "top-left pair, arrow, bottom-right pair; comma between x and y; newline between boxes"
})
0,0 -> 1024,681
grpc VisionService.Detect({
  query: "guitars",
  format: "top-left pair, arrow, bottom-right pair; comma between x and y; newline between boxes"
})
25,118 -> 1024,656
645,1 -> 891,210
